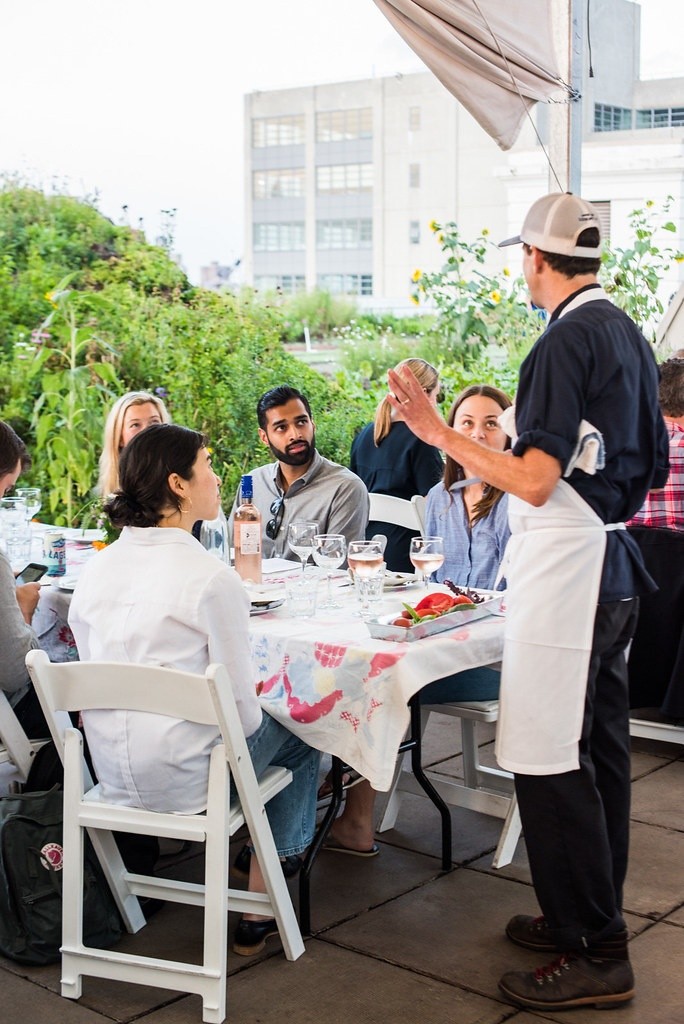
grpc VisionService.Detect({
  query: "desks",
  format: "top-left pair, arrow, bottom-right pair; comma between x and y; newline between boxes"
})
29,522 -> 506,936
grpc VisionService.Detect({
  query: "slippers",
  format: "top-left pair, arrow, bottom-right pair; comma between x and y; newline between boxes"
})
313,826 -> 379,857
315,762 -> 369,811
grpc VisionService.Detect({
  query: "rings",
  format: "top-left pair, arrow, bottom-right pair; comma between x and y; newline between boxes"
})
402,398 -> 409,405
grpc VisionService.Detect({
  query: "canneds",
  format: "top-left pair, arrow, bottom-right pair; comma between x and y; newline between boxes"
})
44,530 -> 66,576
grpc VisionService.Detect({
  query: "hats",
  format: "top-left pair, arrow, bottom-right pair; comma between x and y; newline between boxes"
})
498,191 -> 603,259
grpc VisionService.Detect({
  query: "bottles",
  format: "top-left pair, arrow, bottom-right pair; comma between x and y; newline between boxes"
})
233,475 -> 263,585
199,507 -> 230,568
228,488 -> 238,570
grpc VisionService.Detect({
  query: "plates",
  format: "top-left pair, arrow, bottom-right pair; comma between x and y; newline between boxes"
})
63,528 -> 108,544
56,577 -> 77,591
364,595 -> 505,644
250,600 -> 286,617
383,585 -> 410,593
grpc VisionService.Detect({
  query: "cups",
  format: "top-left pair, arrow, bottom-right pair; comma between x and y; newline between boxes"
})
353,560 -> 387,617
6,527 -> 32,568
285,573 -> 319,620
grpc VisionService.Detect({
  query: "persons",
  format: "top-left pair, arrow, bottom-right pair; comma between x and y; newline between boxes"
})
68,422 -> 319,957
386,191 -> 671,1007
620,357 -> 684,531
226,386 -> 370,570
321,385 -> 514,855
349,358 -> 446,573
96,391 -> 227,551
0,421 -> 161,913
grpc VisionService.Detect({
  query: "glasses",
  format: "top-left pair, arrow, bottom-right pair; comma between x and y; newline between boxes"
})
265,498 -> 285,540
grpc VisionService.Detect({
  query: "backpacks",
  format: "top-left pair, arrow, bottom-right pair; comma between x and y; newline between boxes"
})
0,781 -> 124,967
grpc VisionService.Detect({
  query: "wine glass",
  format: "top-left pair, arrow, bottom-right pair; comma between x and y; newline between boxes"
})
347,540 -> 383,619
287,522 -> 320,586
14,488 -> 41,526
0,496 -> 26,538
409,535 -> 445,596
312,533 -> 346,609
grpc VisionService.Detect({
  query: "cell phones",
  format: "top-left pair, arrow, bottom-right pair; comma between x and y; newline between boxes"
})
14,563 -> 47,584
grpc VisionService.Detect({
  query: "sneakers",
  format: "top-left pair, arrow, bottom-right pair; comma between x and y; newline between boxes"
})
498,952 -> 636,1011
506,913 -> 629,954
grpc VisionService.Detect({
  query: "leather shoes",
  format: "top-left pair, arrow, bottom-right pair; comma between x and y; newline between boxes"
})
230,902 -> 296,958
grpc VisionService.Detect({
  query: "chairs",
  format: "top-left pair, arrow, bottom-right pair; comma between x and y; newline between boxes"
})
376,699 -> 522,869
0,686 -> 50,784
25,648 -> 306,1024
368,493 -> 425,536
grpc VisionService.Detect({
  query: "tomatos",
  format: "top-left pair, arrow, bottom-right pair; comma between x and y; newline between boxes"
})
392,592 -> 474,627
